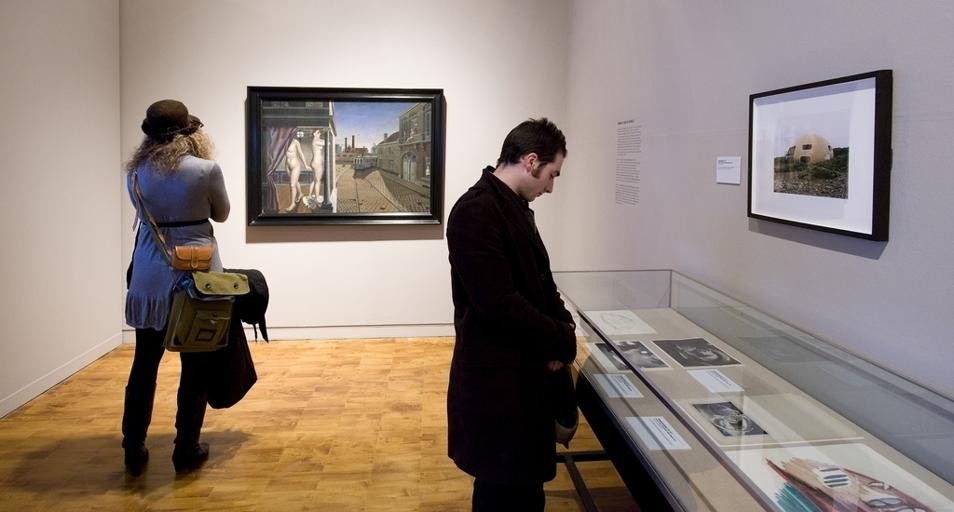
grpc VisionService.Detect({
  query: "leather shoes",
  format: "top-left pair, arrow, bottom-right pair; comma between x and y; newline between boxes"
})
124,444 -> 150,476
172,443 -> 210,470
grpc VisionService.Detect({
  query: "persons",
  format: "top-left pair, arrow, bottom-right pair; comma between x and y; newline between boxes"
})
306,129 -> 330,204
444,116 -> 578,512
116,99 -> 230,486
282,137 -> 309,213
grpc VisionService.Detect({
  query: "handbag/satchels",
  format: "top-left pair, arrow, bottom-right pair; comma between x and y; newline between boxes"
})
159,267 -> 251,355
201,315 -> 258,410
228,262 -> 272,343
129,167 -> 213,273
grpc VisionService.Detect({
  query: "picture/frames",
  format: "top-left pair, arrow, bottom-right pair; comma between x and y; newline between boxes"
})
745,68 -> 896,248
241,81 -> 447,231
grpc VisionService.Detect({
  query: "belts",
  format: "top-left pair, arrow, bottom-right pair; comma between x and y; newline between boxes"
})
157,218 -> 209,230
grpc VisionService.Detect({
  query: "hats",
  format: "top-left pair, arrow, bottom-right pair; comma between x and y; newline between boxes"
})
141,98 -> 202,141
